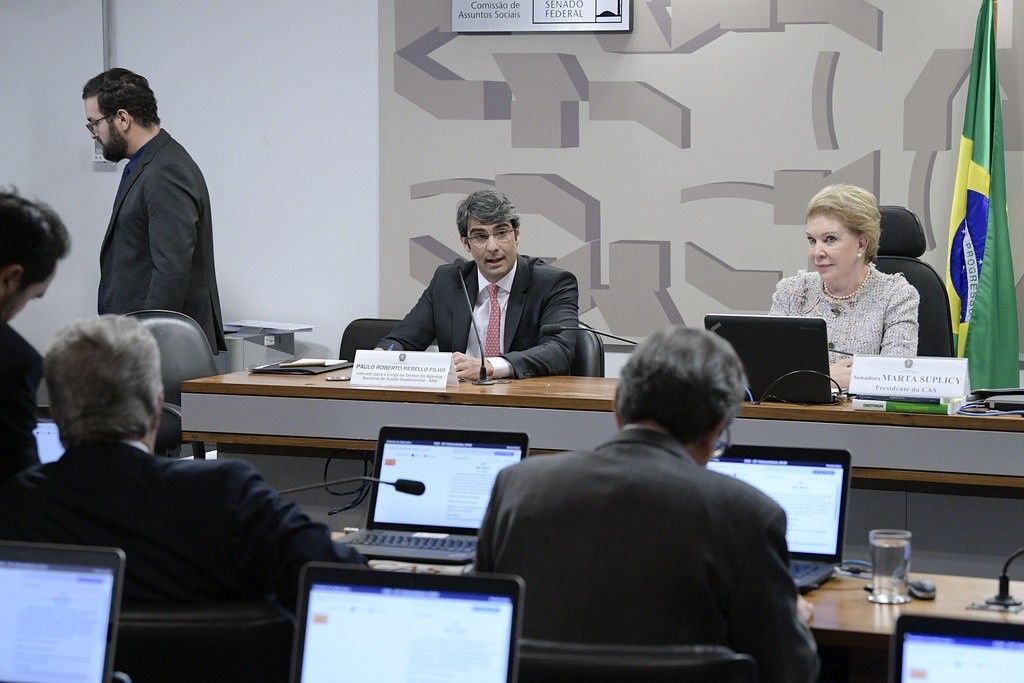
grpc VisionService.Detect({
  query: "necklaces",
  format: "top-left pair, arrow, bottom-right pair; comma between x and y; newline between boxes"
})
823,265 -> 871,300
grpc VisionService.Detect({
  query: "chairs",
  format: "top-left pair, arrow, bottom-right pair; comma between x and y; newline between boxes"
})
874,205 -> 955,357
520,635 -> 759,683
572,328 -> 604,379
118,307 -> 220,462
115,592 -> 296,683
338,318 -> 402,364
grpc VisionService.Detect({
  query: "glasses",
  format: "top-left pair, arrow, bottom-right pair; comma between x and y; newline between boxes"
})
86,112 -> 118,135
712,426 -> 732,458
467,229 -> 515,247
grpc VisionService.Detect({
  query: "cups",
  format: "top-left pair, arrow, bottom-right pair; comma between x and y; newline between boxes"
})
870,529 -> 912,599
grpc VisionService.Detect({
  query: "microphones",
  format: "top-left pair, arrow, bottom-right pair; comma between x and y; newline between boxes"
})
539,325 -> 638,345
453,258 -> 495,385
273,475 -> 426,496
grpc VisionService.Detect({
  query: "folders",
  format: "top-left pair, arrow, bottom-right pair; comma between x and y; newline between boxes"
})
247,359 -> 354,375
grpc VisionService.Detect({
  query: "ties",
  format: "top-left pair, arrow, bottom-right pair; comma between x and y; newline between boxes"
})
485,283 -> 501,357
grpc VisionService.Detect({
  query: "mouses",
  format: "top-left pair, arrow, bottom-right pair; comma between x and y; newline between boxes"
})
906,579 -> 937,600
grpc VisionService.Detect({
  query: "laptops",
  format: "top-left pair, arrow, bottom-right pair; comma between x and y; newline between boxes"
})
331,426 -> 531,566
30,419 -> 67,464
0,541 -> 127,682
289,562 -> 525,683
704,315 -> 849,406
702,444 -> 851,598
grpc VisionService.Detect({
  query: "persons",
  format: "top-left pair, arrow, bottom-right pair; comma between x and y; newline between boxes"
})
80,67 -> 229,461
768,182 -> 920,393
373,189 -> 580,380
473,325 -> 821,683
0,313 -> 368,683
0,194 -> 73,487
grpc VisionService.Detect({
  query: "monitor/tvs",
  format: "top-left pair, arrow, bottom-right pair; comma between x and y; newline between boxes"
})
890,612 -> 1024,683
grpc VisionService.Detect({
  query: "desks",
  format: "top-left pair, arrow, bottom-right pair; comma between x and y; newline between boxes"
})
314,521 -> 1024,683
177,357 -> 1024,539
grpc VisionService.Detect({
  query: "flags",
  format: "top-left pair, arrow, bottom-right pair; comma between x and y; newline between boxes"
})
946,0 -> 1020,397
